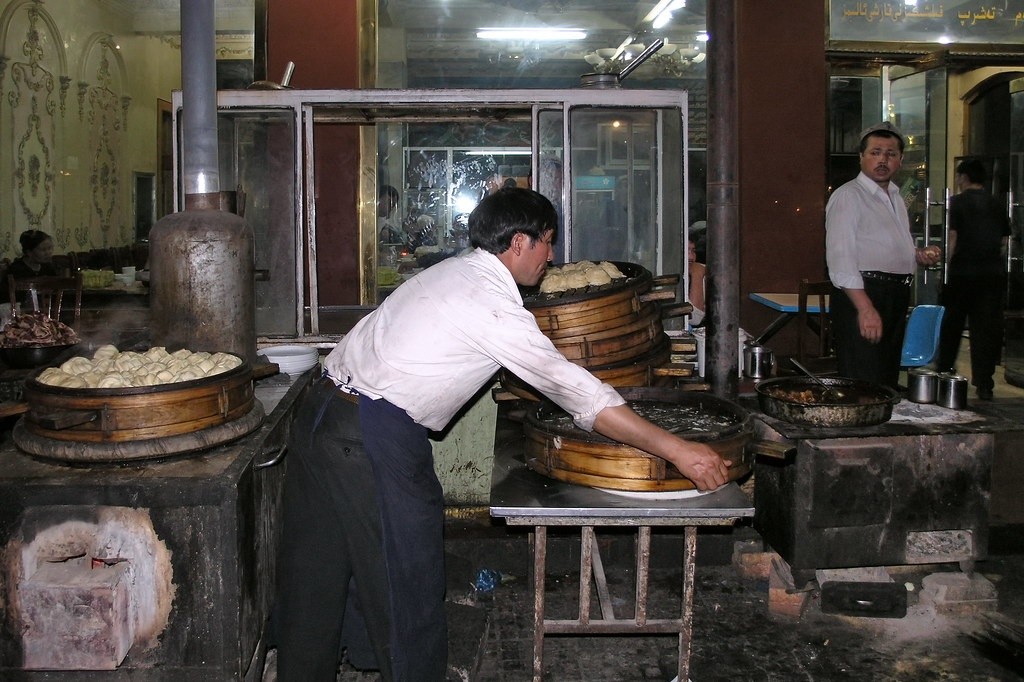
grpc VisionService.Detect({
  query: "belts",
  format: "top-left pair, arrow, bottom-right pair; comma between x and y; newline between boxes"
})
859,271 -> 914,286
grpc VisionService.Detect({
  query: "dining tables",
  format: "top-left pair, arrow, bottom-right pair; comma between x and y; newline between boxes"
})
65,277 -> 144,333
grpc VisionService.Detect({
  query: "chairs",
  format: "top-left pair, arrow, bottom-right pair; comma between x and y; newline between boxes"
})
8,273 -> 82,337
70,244 -> 149,273
899,305 -> 946,367
772,278 -> 837,376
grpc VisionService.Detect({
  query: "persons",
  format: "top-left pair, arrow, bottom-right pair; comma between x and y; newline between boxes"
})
0,230 -> 61,304
378,185 -> 406,244
410,207 -> 434,231
824,129 -> 941,387
274,183 -> 733,682
688,234 -> 707,325
936,159 -> 1011,402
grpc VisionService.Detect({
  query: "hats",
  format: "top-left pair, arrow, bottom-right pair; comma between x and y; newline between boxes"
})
957,160 -> 987,186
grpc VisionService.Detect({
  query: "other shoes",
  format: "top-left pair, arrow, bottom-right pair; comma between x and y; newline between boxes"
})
976,389 -> 993,399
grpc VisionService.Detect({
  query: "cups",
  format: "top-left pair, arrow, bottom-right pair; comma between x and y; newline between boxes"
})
937,368 -> 968,410
907,367 -> 937,404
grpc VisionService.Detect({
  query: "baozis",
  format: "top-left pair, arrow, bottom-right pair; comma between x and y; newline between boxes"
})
538,259 -> 623,293
37,344 -> 242,388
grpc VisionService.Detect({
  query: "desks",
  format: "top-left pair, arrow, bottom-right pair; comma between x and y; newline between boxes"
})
490,419 -> 755,682
749,292 -> 834,347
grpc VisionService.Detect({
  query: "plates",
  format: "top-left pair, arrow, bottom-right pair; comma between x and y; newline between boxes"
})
257,345 -> 319,375
